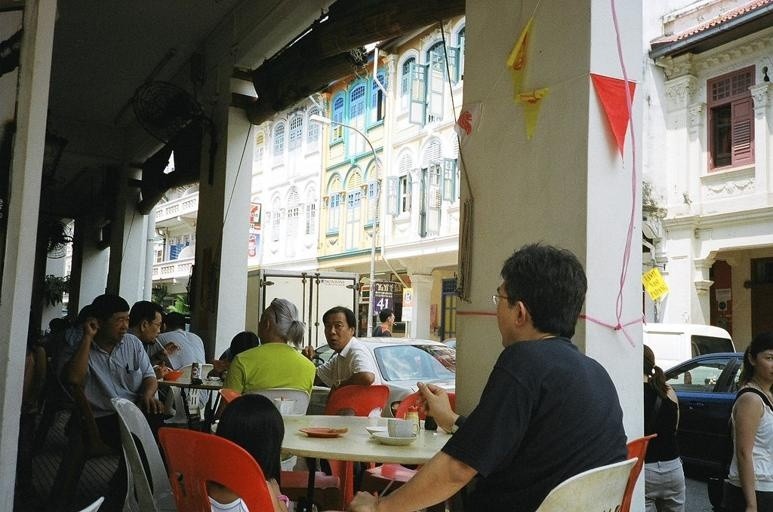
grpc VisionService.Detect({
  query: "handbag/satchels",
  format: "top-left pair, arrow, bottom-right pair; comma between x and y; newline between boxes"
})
706,474 -> 727,511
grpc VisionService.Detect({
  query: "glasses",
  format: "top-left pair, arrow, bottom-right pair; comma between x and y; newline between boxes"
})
150,321 -> 162,329
269,296 -> 279,324
489,294 -> 529,313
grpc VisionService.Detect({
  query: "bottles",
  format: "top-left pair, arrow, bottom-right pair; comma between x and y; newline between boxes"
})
405,406 -> 420,434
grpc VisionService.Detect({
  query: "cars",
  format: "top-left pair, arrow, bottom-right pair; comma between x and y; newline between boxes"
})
656,351 -> 749,481
305,342 -> 458,413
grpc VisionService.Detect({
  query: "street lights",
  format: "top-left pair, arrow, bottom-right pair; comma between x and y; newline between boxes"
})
308,109 -> 379,333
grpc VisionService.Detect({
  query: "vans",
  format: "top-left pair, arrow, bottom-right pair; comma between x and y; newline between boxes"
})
642,321 -> 734,371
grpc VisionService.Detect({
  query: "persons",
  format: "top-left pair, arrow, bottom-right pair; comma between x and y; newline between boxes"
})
721,332 -> 773,511
48,294 -> 209,443
350,241 -> 630,511
301,303 -> 386,421
371,308 -> 396,337
643,344 -> 685,511
209,299 -> 316,399
182,393 -> 287,510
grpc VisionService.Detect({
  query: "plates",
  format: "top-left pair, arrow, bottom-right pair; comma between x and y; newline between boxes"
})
298,426 -> 344,439
364,425 -> 416,445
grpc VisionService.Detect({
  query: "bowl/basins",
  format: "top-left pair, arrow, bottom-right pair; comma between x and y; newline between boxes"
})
161,369 -> 182,381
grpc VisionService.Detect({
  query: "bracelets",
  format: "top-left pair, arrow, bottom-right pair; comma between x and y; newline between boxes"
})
449,414 -> 468,437
276,493 -> 291,508
334,377 -> 342,388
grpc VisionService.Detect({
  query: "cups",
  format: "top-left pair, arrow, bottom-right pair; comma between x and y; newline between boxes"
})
387,419 -> 418,437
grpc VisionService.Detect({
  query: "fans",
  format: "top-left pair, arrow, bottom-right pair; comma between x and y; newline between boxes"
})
37,218 -> 76,258
130,81 -> 217,151
